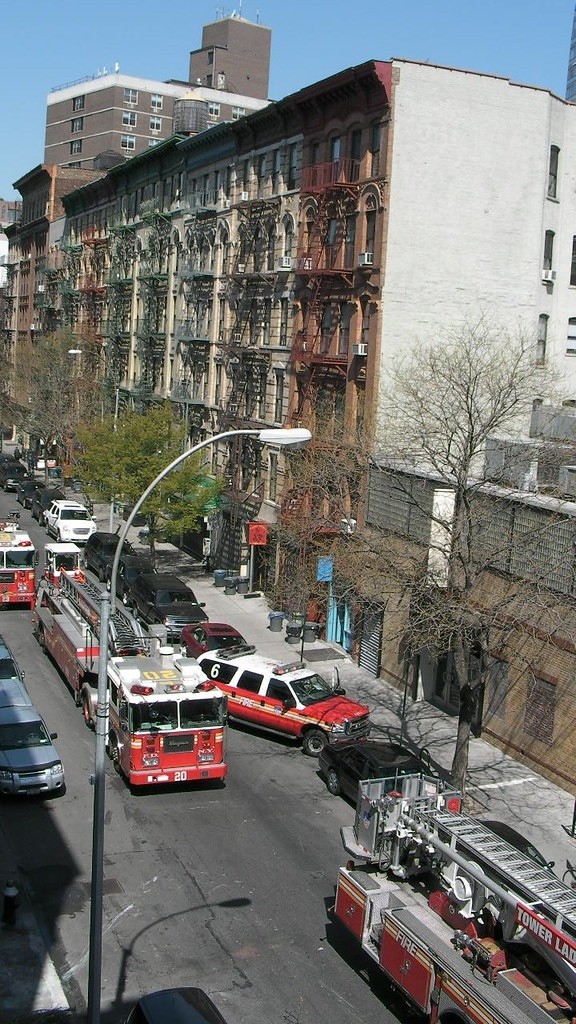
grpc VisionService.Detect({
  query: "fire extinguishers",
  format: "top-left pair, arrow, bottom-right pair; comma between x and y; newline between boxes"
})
193,680 -> 216,693
130,684 -> 154,696
18,540 -> 31,547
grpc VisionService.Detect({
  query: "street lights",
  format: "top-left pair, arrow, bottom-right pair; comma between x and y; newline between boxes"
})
84,425 -> 314,1022
69,347 -> 119,534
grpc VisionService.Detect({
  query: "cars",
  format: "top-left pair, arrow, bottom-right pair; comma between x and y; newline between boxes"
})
319,740 -> 434,806
16,481 -> 47,509
181,625 -> 249,659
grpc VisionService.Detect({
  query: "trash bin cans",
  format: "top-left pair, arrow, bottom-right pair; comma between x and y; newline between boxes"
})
236,575 -> 250,594
286,622 -> 302,644
268,611 -> 285,632
303,621 -> 318,643
223,576 -> 237,595
213,569 -> 227,587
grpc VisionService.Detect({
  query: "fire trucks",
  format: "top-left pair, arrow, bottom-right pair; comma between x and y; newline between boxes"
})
331,771 -> 576,1024
31,541 -> 230,786
0,510 -> 38,609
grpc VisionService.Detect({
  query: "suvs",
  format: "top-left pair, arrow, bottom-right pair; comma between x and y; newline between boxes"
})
105,555 -> 158,607
0,632 -> 27,690
30,486 -> 66,525
125,573 -> 210,641
40,499 -> 97,543
83,531 -> 137,582
0,676 -> 65,802
0,452 -> 33,493
197,644 -> 372,757
